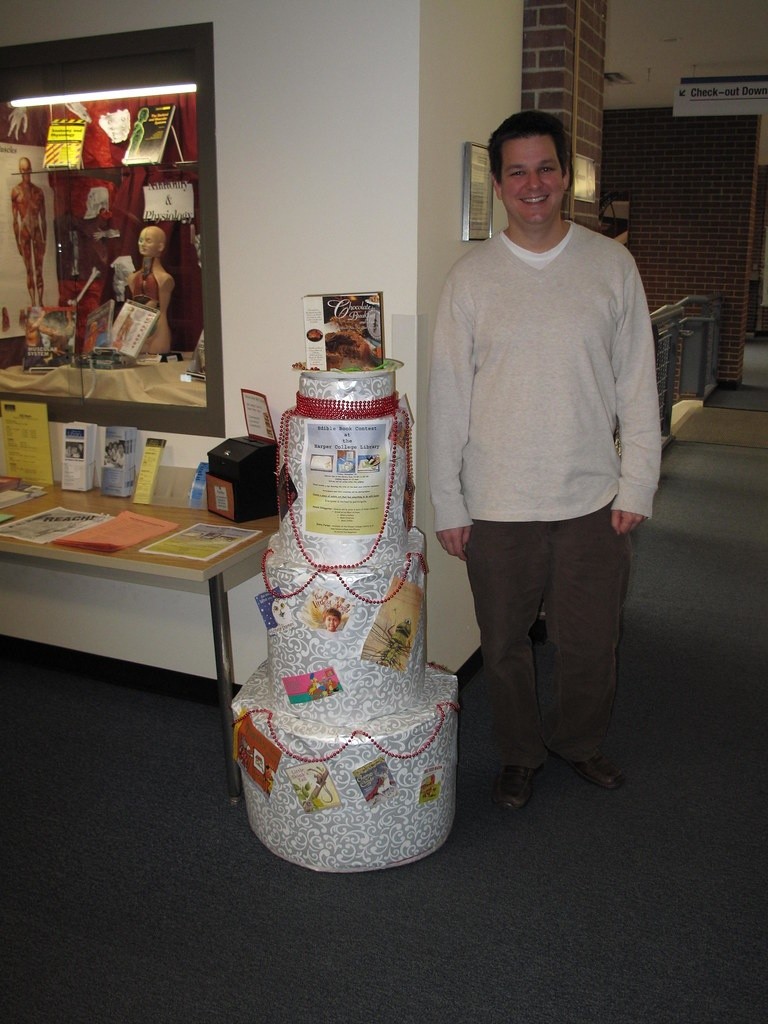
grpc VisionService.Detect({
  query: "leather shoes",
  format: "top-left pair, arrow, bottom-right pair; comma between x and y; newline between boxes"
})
491,763 -> 544,811
548,748 -> 625,790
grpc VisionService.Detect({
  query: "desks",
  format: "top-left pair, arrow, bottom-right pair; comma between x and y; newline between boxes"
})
0,481 -> 286,797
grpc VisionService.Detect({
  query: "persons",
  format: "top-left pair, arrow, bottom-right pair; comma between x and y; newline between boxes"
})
428,111 -> 662,814
127,226 -> 174,355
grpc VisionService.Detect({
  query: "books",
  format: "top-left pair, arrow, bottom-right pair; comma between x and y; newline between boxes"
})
42,119 -> 86,169
24,306 -> 77,373
123,103 -> 176,166
301,292 -> 383,371
84,300 -> 114,352
112,299 -> 161,357
